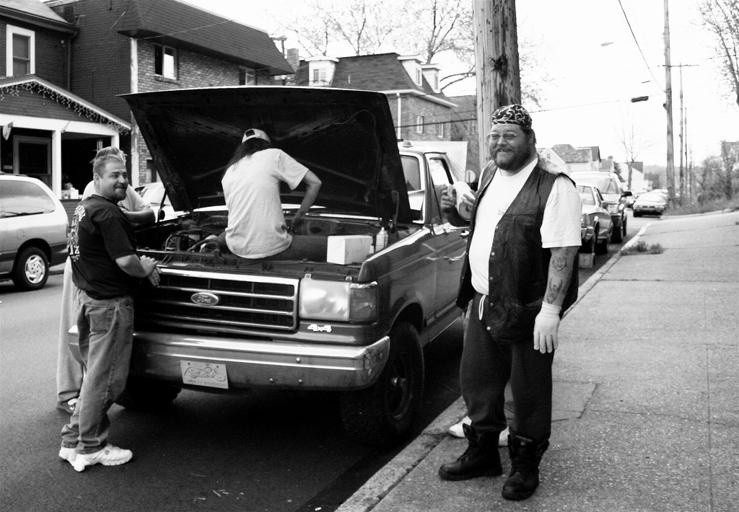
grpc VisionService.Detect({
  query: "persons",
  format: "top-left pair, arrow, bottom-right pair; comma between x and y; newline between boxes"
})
58,154 -> 161,473
438,103 -> 582,502
58,148 -> 155,415
220,129 -> 323,262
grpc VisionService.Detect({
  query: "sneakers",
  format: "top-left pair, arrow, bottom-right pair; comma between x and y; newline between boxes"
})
449,408 -> 539,446
56,396 -> 80,415
56,438 -> 135,474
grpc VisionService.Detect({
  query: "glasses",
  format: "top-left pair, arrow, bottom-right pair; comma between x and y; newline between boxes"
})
485,131 -> 531,142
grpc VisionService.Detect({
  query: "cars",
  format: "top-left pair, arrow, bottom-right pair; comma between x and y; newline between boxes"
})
0,173 -> 71,292
110,85 -> 473,443
560,169 -> 670,258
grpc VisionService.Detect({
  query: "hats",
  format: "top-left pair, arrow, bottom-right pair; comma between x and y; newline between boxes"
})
240,127 -> 270,143
489,103 -> 534,128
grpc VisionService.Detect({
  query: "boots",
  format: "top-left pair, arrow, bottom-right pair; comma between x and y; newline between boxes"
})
500,431 -> 550,502
438,423 -> 504,480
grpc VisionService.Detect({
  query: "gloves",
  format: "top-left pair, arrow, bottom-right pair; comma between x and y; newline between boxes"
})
533,300 -> 562,355
